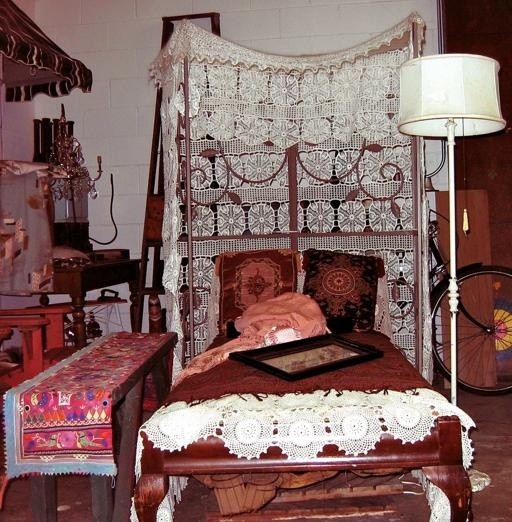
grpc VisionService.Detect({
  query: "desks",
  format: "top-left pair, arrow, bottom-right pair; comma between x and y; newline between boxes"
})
51,252 -> 141,332
1,330 -> 177,522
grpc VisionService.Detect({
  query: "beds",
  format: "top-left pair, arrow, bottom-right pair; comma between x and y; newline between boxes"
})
130,14 -> 474,522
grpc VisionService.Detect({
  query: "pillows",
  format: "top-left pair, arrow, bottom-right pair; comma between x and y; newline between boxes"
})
303,246 -> 391,334
214,245 -> 302,334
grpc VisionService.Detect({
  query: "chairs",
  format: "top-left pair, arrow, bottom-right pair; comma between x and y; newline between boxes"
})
0,302 -> 80,388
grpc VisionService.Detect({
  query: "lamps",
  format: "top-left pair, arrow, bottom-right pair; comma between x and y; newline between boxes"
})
398,50 -> 506,406
45,104 -> 103,202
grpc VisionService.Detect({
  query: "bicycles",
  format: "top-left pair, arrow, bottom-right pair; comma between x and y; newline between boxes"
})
428,210 -> 512,394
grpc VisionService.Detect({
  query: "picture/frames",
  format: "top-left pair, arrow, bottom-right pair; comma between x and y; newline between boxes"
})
228,335 -> 383,385
